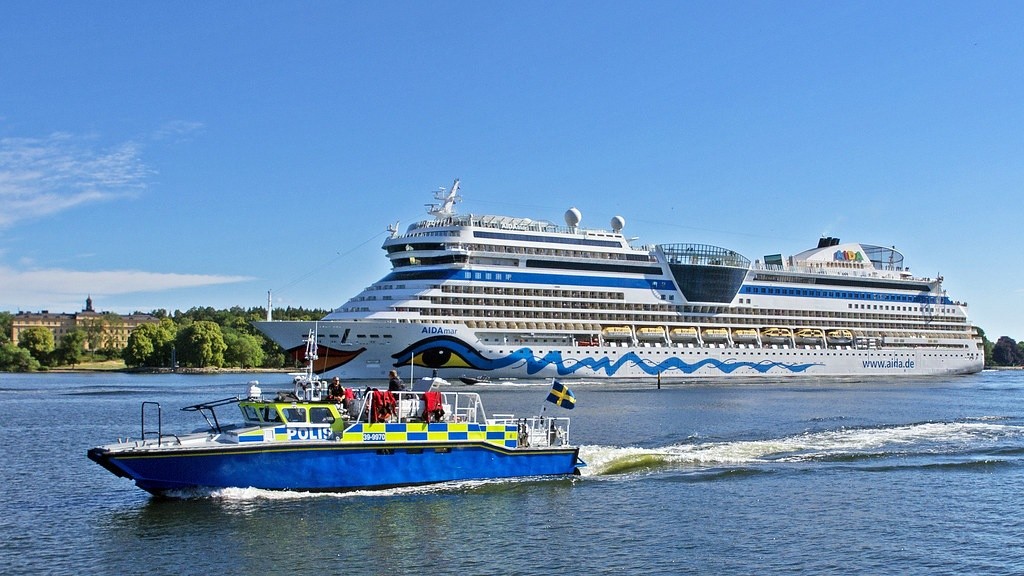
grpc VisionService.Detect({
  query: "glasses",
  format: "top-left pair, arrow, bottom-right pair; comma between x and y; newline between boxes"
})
335,380 -> 339,381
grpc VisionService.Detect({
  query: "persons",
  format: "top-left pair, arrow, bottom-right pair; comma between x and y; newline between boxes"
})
388,370 -> 406,399
327,377 -> 347,415
272,411 -> 288,422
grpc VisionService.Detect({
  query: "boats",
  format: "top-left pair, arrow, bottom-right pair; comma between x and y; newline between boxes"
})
762,329 -> 790,343
636,326 -> 665,341
602,325 -> 632,339
670,328 -> 697,340
87,320 -> 587,498
732,328 -> 758,341
702,328 -> 728,342
826,330 -> 853,343
794,329 -> 823,342
579,336 -> 599,346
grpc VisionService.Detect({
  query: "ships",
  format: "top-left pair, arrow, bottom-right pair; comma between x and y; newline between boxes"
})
250,180 -> 985,382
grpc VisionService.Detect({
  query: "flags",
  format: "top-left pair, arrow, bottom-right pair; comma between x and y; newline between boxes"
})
547,381 -> 576,410
